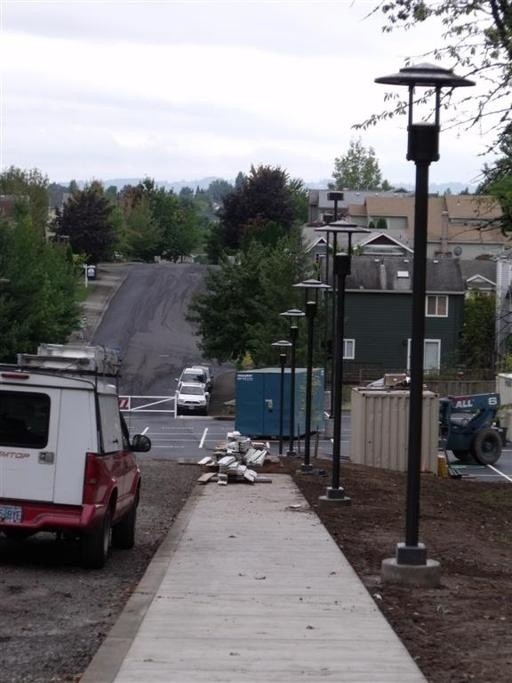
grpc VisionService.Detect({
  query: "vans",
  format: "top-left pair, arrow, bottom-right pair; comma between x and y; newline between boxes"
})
1,363 -> 153,570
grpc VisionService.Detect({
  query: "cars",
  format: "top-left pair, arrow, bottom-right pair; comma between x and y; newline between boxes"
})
174,363 -> 214,414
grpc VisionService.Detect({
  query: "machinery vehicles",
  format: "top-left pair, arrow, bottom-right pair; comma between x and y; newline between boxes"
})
368,371 -> 507,465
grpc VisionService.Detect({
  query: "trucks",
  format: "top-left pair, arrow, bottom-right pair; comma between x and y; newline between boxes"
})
18,342 -> 120,382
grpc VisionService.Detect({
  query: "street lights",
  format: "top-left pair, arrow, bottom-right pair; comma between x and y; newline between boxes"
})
373,62 -> 476,565
269,220 -> 371,498
